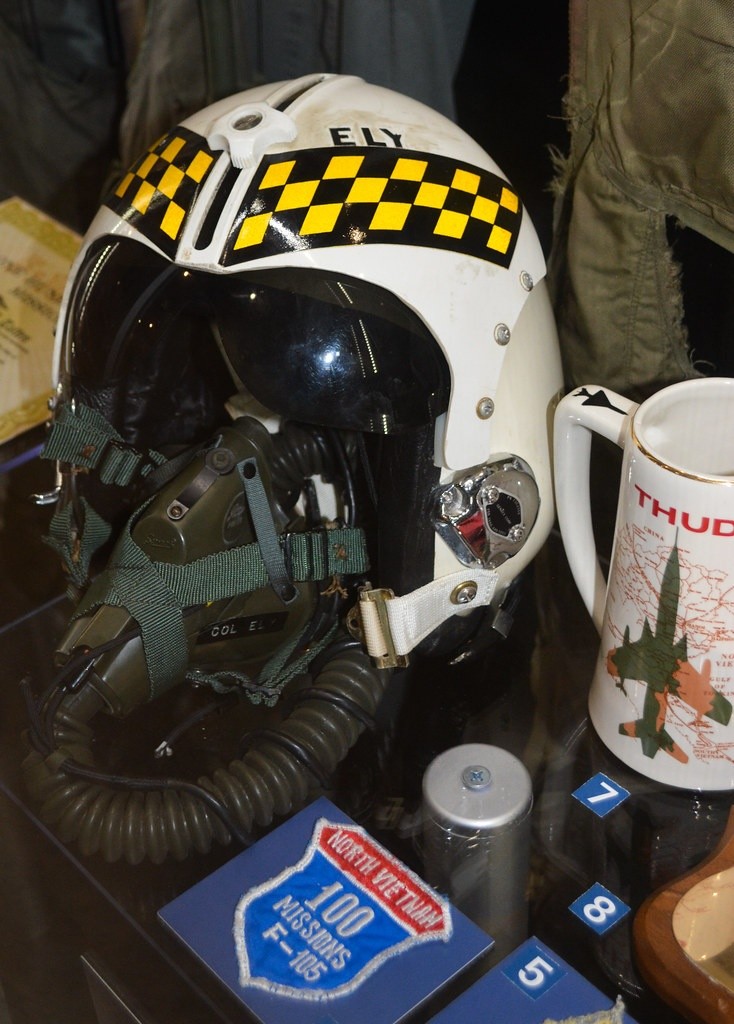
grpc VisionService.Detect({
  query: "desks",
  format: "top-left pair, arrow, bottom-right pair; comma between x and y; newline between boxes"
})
0,420 -> 734,1024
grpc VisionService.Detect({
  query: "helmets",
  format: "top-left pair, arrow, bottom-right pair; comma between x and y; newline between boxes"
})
32,74 -> 568,671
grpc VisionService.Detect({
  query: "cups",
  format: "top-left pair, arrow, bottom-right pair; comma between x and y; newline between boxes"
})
553,376 -> 734,792
